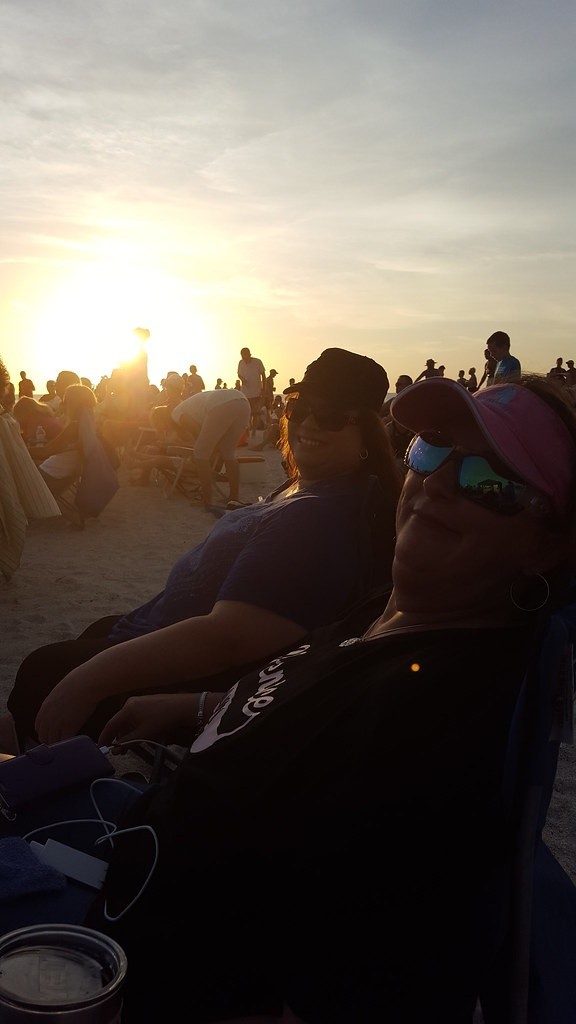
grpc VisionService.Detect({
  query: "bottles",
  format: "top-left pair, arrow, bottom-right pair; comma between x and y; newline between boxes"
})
36,426 -> 46,447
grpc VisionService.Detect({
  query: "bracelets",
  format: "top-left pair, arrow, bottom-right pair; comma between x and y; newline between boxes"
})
198,692 -> 209,721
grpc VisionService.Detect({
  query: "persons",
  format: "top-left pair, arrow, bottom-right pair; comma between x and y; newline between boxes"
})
477,349 -> 498,390
237,348 -> 295,451
150,388 -> 251,507
414,359 -> 445,382
487,331 -> 521,385
396,375 -> 412,394
467,368 -> 477,392
549,358 -> 576,384
456,370 -> 467,387
0,360 -> 62,580
6,347 -> 405,757
112,328 -> 151,417
0,376 -> 576,1024
4,365 -> 241,509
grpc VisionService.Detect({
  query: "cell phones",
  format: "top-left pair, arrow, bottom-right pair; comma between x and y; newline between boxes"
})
37,838 -> 109,891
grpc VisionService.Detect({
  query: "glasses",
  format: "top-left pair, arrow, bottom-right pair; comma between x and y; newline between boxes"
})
404,430 -> 536,516
282,398 -> 353,433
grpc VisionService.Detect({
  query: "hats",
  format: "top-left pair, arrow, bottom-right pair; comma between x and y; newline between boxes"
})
281,347 -> 389,415
389,376 -> 576,521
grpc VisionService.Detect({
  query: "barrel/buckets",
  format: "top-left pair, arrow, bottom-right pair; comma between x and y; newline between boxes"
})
0,922 -> 128,1024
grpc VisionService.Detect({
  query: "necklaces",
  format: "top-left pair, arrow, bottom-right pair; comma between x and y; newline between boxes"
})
339,617 -> 428,647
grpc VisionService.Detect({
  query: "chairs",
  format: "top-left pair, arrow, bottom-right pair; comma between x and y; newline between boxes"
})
136,426 -> 225,498
37,450 -> 111,530
